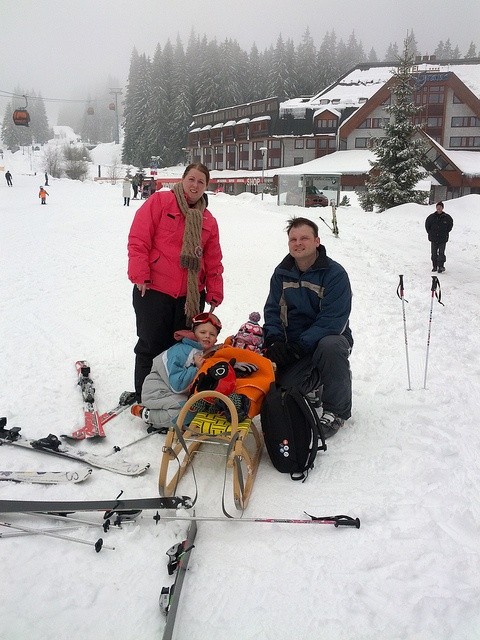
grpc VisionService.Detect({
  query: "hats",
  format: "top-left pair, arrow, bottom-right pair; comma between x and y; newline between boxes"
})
234,312 -> 265,349
436,200 -> 444,208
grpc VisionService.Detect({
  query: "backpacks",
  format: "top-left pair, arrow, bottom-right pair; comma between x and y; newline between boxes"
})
260,381 -> 327,483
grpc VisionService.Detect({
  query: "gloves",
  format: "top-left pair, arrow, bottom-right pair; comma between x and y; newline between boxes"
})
233,362 -> 259,375
265,334 -> 294,373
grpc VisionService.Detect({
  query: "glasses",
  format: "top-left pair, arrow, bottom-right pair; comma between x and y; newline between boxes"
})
191,313 -> 222,335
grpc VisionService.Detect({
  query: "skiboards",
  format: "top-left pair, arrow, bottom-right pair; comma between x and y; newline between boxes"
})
60,360 -> 137,441
0,496 -> 197,640
0,428 -> 150,485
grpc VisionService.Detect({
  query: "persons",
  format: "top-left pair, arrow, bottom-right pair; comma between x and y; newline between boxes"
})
130,313 -> 222,430
38,186 -> 49,204
123,177 -> 131,206
147,177 -> 157,199
5,171 -> 12,186
424,202 -> 453,273
186,312 -> 266,422
126,162 -> 223,404
44,173 -> 49,186
132,173 -> 140,200
263,216 -> 354,441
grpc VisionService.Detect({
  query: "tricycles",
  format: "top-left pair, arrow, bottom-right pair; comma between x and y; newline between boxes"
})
138,177 -> 157,199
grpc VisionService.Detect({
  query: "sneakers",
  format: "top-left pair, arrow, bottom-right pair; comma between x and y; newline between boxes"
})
438,267 -> 445,273
228,393 -> 241,410
131,404 -> 145,418
306,384 -> 323,408
432,267 -> 437,271
318,411 -> 344,439
189,394 -> 219,414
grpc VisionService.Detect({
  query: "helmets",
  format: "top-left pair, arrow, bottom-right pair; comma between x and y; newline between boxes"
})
190,360 -> 236,404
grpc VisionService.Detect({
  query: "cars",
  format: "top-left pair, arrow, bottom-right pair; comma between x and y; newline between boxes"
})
286,186 -> 328,206
